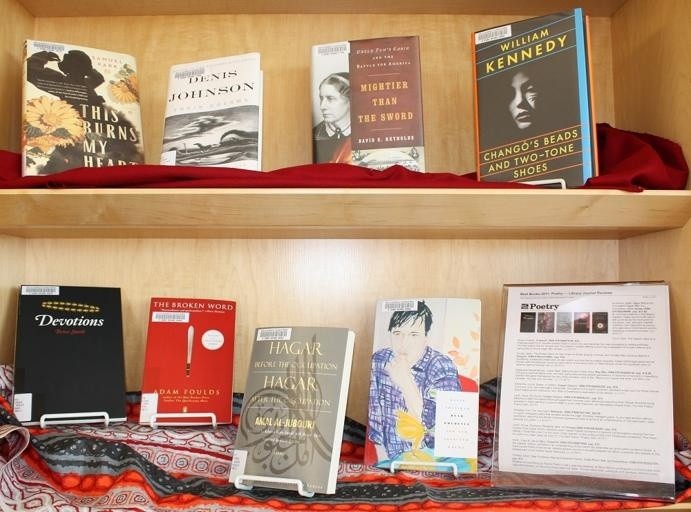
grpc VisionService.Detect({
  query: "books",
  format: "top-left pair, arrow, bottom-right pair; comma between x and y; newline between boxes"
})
469,6 -> 602,191
20,32 -> 145,179
12,282 -> 129,429
158,53 -> 266,172
227,325 -> 356,496
364,294 -> 480,475
136,296 -> 237,430
311,37 -> 424,178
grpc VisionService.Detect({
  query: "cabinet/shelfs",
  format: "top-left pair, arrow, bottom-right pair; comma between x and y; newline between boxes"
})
0,0 -> 690,511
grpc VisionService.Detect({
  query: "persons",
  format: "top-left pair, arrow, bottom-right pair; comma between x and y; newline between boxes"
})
498,66 -> 549,139
312,71 -> 353,166
366,302 -> 466,471
